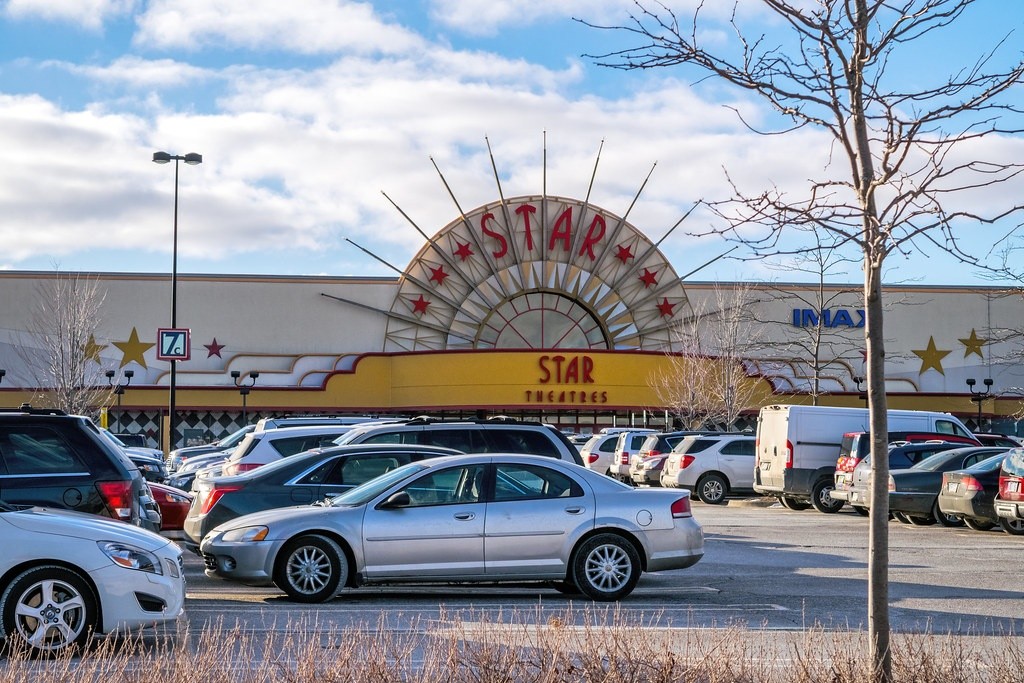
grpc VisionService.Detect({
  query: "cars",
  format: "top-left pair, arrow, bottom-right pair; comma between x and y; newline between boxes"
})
0,501 -> 186,657
862,446 -> 1016,527
98,411 -> 669,529
938,451 -> 1024,535
200,452 -> 707,603
183,444 -> 548,580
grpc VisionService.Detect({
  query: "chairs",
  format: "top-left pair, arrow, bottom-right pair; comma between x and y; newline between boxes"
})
467,470 -> 483,503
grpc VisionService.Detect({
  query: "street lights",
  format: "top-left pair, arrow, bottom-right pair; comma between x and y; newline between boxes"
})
852,376 -> 870,409
230,370 -> 260,427
965,378 -> 994,433
106,370 -> 135,434
151,150 -> 202,453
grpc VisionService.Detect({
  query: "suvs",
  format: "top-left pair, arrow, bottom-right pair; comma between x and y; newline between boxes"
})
850,440 -> 979,528
316,414 -> 584,468
660,435 -> 767,506
974,432 -> 1024,448
993,446 -> 1024,536
0,402 -> 163,535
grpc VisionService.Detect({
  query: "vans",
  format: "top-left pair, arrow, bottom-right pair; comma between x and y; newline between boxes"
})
830,430 -> 983,525
753,404 -> 983,513
